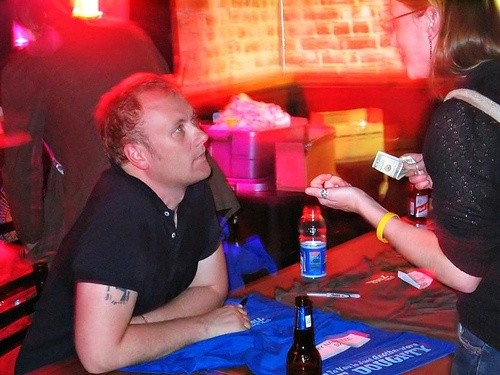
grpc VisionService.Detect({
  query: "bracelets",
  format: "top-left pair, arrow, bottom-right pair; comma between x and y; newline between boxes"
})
376,212 -> 400,243
139,313 -> 148,324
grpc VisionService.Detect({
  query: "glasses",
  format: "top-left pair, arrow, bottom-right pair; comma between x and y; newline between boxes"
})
380,7 -> 427,33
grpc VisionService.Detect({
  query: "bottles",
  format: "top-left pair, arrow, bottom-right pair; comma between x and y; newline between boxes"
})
406,178 -> 429,224
298,195 -> 328,282
33,262 -> 50,301
286,296 -> 321,375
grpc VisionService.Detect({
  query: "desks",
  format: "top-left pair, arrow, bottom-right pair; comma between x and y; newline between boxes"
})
201,159 -> 406,262
29,219 -> 499,374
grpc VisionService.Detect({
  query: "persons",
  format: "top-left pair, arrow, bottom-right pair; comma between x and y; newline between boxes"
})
15,71 -> 251,375
0,0 -> 242,270
305,0 -> 500,375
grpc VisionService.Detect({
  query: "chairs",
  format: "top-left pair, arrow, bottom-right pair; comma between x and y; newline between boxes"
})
0,260 -> 48,366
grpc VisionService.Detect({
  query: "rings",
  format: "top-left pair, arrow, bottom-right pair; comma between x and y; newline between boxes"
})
321,188 -> 328,199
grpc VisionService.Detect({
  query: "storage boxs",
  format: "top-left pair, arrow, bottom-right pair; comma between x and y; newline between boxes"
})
199,107 -> 384,199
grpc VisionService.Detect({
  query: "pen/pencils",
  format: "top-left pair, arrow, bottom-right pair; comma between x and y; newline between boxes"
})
306,291 -> 361,300
236,293 -> 249,309
